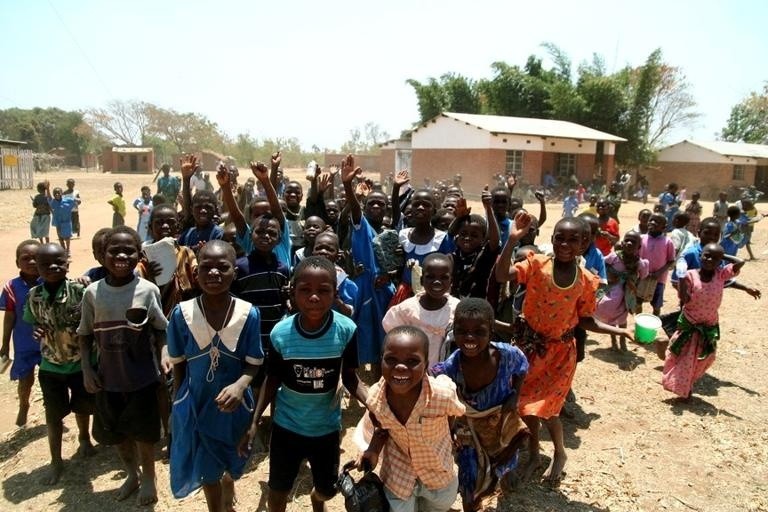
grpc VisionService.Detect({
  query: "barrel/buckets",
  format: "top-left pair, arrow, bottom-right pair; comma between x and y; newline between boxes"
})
634,311 -> 663,344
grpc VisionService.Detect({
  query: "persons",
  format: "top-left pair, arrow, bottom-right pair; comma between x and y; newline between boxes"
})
2,152 -> 603,487
356,327 -> 473,510
541,168 -> 768,399
236,255 -> 379,512
161,239 -> 263,512
427,298 -> 527,512
